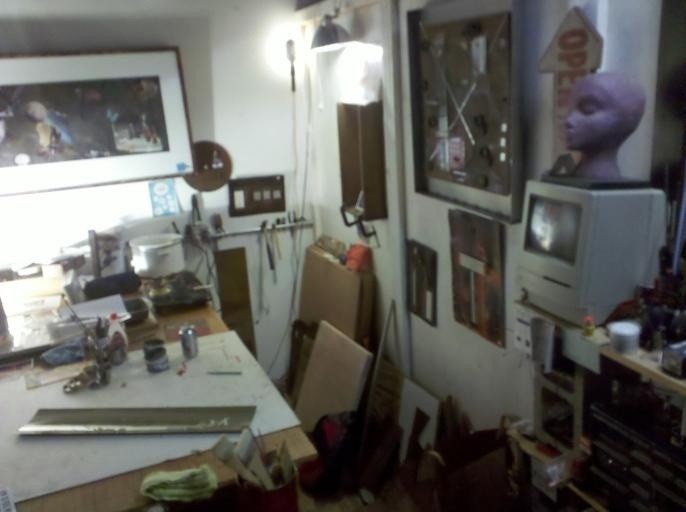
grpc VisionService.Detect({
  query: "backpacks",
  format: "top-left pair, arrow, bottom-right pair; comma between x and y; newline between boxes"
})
298,412 -> 370,499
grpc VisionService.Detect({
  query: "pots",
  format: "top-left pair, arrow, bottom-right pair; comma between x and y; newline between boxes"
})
130,232 -> 185,278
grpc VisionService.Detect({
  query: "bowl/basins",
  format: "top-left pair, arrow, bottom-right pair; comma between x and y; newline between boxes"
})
148,279 -> 177,305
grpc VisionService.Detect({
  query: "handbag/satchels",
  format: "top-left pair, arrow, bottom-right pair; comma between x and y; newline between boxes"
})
436,429 -> 523,512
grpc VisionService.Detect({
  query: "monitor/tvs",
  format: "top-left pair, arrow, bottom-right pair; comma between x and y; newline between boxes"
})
516,180 -> 667,326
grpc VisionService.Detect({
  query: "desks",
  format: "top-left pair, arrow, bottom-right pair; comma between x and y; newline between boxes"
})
0,255 -> 318,511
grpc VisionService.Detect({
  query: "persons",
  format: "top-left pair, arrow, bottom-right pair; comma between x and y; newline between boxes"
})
561,70 -> 646,180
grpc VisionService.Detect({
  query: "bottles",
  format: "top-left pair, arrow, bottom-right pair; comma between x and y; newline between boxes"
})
104,313 -> 129,367
178,324 -> 200,358
287,210 -> 297,224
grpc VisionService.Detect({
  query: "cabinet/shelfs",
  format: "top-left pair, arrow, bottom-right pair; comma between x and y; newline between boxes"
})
506,300 -> 686,511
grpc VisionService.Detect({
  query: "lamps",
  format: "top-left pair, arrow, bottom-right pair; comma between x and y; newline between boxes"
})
310,8 -> 354,54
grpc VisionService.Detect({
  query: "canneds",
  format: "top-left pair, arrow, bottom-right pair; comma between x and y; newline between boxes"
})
142,339 -> 170,374
177,325 -> 198,360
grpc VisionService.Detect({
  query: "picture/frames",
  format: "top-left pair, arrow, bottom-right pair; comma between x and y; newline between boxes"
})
406,239 -> 437,328
1,46 -> 199,196
407,1 -> 522,225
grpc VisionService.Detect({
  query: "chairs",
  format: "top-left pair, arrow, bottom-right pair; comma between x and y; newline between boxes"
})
294,320 -> 374,433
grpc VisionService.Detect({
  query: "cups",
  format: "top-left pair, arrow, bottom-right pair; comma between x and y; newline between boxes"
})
605,321 -> 641,356
